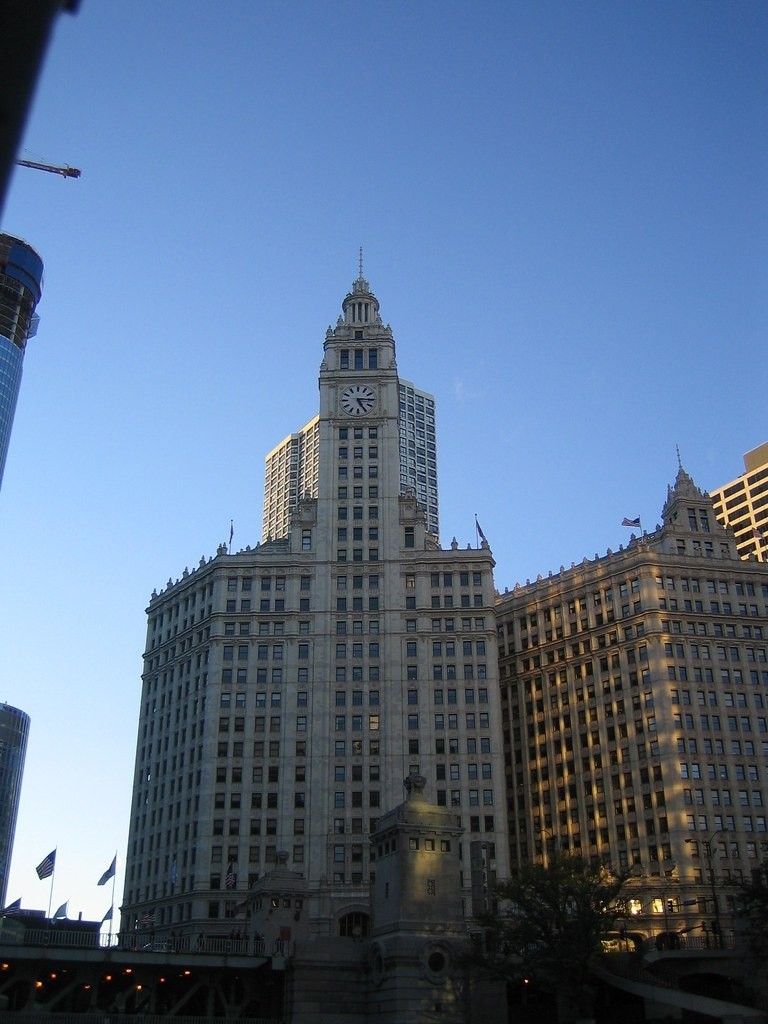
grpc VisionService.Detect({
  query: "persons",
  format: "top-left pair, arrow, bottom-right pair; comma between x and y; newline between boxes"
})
169,928 -> 285,957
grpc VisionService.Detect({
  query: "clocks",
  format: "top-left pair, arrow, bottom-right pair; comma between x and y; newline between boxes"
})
339,384 -> 378,418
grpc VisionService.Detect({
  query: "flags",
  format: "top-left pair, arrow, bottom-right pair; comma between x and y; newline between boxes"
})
51,901 -> 67,922
36,850 -> 56,880
97,854 -> 116,885
233,901 -> 248,916
102,907 -> 112,922
138,907 -> 155,924
0,898 -> 20,916
225,862 -> 235,887
168,862 -> 178,884
